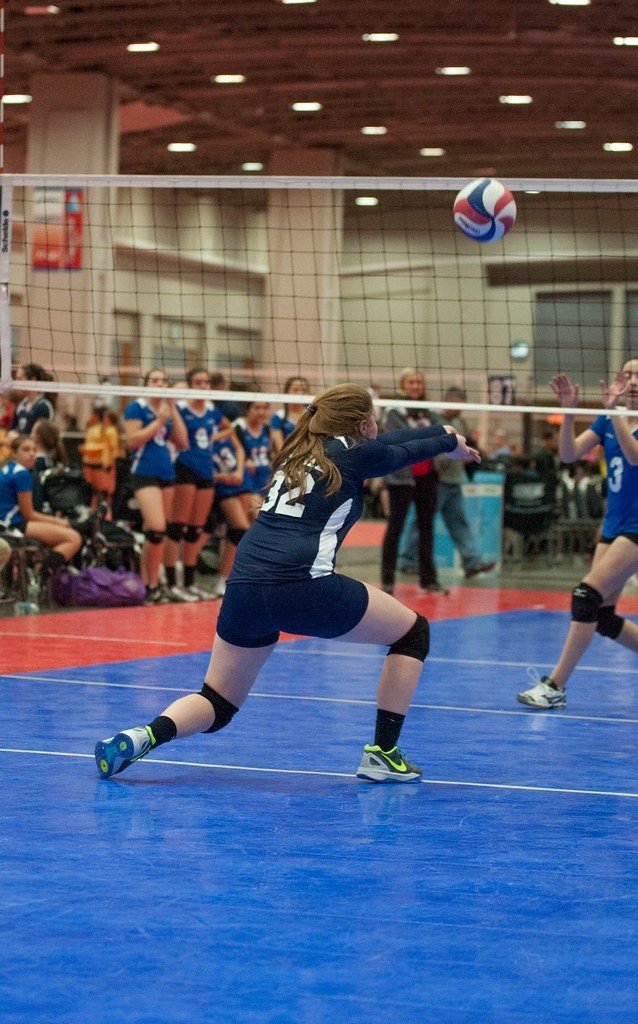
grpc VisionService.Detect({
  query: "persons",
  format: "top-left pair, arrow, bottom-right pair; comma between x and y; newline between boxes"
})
516,355 -> 638,710
94,383 -> 482,783
0,361 -> 609,608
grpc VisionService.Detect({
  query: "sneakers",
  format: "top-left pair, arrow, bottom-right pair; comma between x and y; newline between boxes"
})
517,667 -> 567,709
356,745 -> 422,782
95,725 -> 156,779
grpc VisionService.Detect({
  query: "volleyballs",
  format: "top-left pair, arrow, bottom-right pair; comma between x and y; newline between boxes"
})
453,177 -> 517,243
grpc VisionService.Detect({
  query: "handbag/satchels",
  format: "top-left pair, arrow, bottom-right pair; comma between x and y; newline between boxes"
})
51,566 -> 146,607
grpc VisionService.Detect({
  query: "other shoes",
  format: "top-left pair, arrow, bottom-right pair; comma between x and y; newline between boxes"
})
419,583 -> 450,595
380,584 -> 394,595
464,562 -> 496,579
143,575 -> 228,606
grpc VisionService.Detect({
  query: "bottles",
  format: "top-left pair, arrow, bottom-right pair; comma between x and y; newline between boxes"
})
14,603 -> 39,611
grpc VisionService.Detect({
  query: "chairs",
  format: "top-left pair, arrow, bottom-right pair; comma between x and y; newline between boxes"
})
0,468 -> 141,613
500,454 -> 607,567
